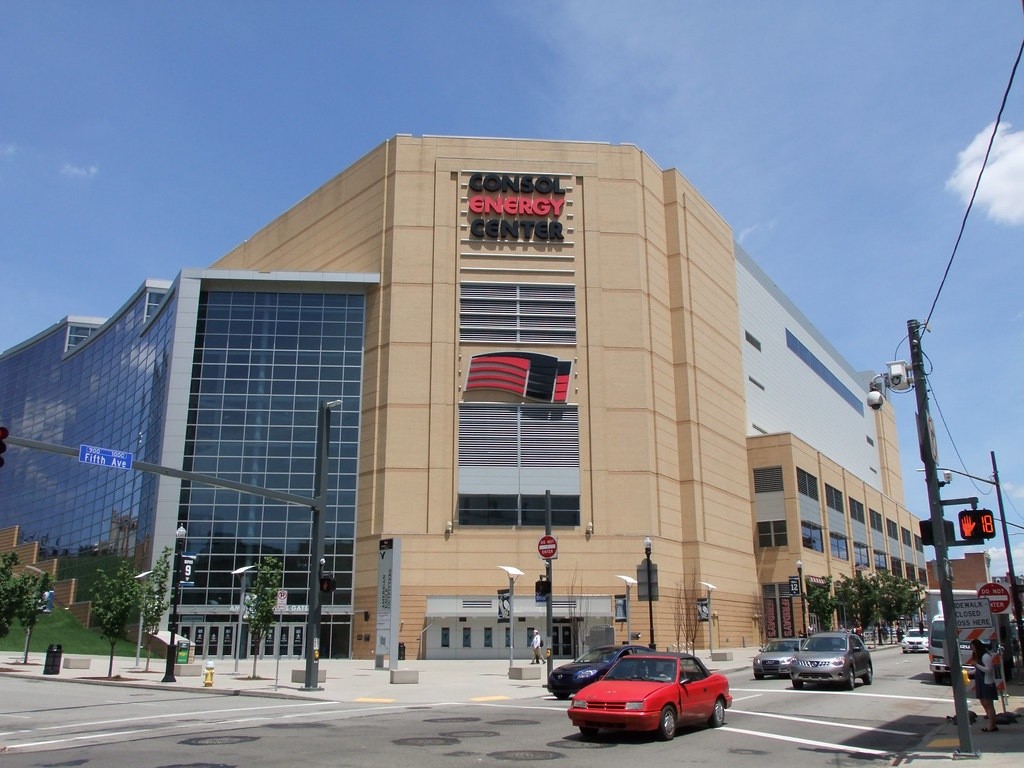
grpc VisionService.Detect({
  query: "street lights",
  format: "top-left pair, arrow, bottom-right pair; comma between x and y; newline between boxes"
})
230,565 -> 257,673
699,581 -> 717,658
871,573 -> 884,646
159,521 -> 188,682
617,575 -> 638,645
795,559 -> 809,638
643,535 -> 657,652
497,565 -> 527,667
915,450 -> 1024,667
134,570 -> 155,666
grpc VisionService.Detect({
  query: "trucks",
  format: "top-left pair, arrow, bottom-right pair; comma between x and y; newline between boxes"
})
923,588 -> 1016,686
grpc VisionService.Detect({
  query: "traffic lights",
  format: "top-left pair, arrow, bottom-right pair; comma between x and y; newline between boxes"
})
535,580 -> 551,596
957,508 -> 996,540
0,425 -> 10,469
321,577 -> 336,593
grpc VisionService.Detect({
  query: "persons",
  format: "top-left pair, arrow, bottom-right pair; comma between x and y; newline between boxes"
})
529,629 -> 546,665
919,620 -> 924,629
873,619 -> 880,629
849,625 -> 856,634
798,629 -> 804,637
895,622 -> 899,633
966,639 -> 998,732
908,624 -> 912,627
662,664 -> 673,679
839,625 -> 844,631
808,623 -> 812,637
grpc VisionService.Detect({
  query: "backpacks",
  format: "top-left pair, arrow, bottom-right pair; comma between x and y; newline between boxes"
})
540,638 -> 543,647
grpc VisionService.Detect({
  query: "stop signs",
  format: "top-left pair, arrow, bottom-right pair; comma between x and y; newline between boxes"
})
537,535 -> 558,558
977,582 -> 1012,614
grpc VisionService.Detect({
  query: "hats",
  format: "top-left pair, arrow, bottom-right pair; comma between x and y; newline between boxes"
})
534,630 -> 538,632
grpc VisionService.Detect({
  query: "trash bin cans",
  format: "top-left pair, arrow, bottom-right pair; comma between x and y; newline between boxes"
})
398,642 -> 406,660
43,643 -> 64,675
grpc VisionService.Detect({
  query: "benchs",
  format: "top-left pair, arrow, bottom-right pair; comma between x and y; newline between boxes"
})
509,666 -> 541,680
711,652 -> 733,661
64,657 -> 91,669
292,670 -> 326,683
174,664 -> 202,676
390,669 -> 419,684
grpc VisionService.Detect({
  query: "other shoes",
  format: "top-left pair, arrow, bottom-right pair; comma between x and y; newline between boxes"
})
529,662 -> 535,664
981,727 -> 1000,732
542,661 -> 546,664
984,715 -> 999,719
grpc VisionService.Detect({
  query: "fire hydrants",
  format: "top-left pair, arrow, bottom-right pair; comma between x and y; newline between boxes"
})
202,658 -> 216,687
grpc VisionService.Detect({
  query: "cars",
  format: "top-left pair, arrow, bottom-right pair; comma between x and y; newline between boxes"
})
565,650 -> 734,742
789,631 -> 874,691
751,638 -> 808,679
547,644 -> 675,700
900,628 -> 929,654
